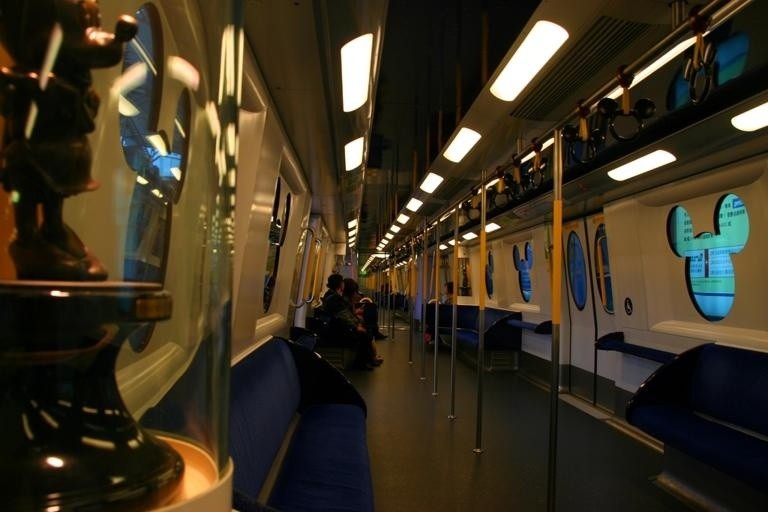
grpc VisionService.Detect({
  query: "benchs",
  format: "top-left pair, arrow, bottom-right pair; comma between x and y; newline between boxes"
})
306,303 -> 378,371
421,303 -> 522,372
230,337 -> 374,512
624,344 -> 768,495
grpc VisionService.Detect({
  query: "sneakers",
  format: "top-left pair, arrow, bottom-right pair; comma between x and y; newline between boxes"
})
353,363 -> 373,371
375,334 -> 388,340
369,355 -> 384,366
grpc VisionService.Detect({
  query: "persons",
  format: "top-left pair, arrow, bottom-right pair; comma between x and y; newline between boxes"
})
428,281 -> 456,345
322,274 -> 388,366
